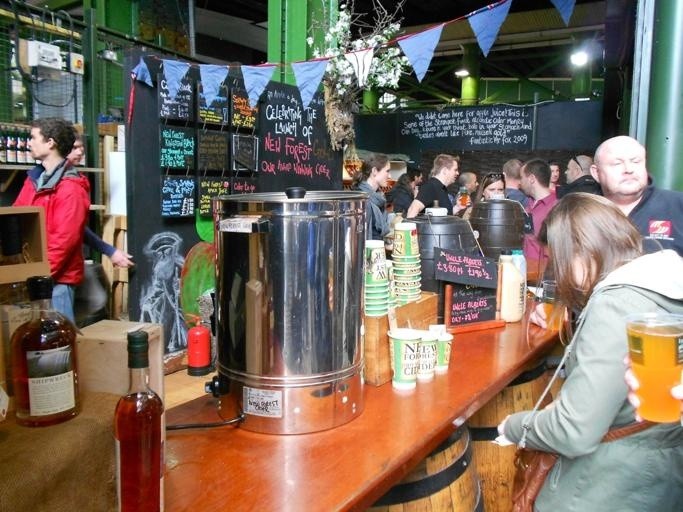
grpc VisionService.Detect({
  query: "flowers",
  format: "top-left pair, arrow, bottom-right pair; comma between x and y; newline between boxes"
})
306,0 -> 434,114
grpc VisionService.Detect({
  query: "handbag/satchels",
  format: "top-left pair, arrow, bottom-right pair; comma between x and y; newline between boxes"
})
512,448 -> 558,512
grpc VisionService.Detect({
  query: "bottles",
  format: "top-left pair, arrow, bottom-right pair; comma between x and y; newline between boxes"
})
391,213 -> 404,224
510,249 -> 530,276
9,273 -> 81,427
498,251 -> 526,326
114,329 -> 166,511
0,123 -> 40,165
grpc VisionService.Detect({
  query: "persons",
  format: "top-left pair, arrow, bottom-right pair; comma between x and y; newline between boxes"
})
9,117 -> 92,335
591,135 -> 683,255
623,347 -> 683,425
496,192 -> 682,512
352,152 -> 603,276
64,133 -> 136,321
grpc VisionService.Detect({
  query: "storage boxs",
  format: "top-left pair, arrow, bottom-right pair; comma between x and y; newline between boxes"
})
366,290 -> 437,387
74,319 -> 164,402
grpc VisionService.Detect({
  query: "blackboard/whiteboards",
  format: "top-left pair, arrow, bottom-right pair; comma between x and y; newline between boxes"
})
433,246 -> 497,289
444,285 -> 506,335
354,102 -> 534,154
157,72 -> 261,218
261,80 -> 343,193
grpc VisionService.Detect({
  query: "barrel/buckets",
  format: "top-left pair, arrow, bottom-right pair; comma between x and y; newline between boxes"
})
468,193 -> 529,262
402,209 -> 479,326
367,424 -> 485,512
465,360 -> 550,512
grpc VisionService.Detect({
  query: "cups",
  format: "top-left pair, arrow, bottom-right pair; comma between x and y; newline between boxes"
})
624,313 -> 682,424
385,328 -> 420,390
435,330 -> 455,369
416,329 -> 438,382
458,186 -> 468,206
363,222 -> 420,317
539,278 -> 564,333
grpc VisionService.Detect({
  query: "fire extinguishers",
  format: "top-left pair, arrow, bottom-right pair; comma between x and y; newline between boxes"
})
185,313 -> 212,376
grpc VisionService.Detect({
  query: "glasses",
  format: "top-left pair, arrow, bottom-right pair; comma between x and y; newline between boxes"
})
572,154 -> 583,173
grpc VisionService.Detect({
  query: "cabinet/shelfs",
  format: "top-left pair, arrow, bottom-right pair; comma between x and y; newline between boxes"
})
0,165 -> 107,210
